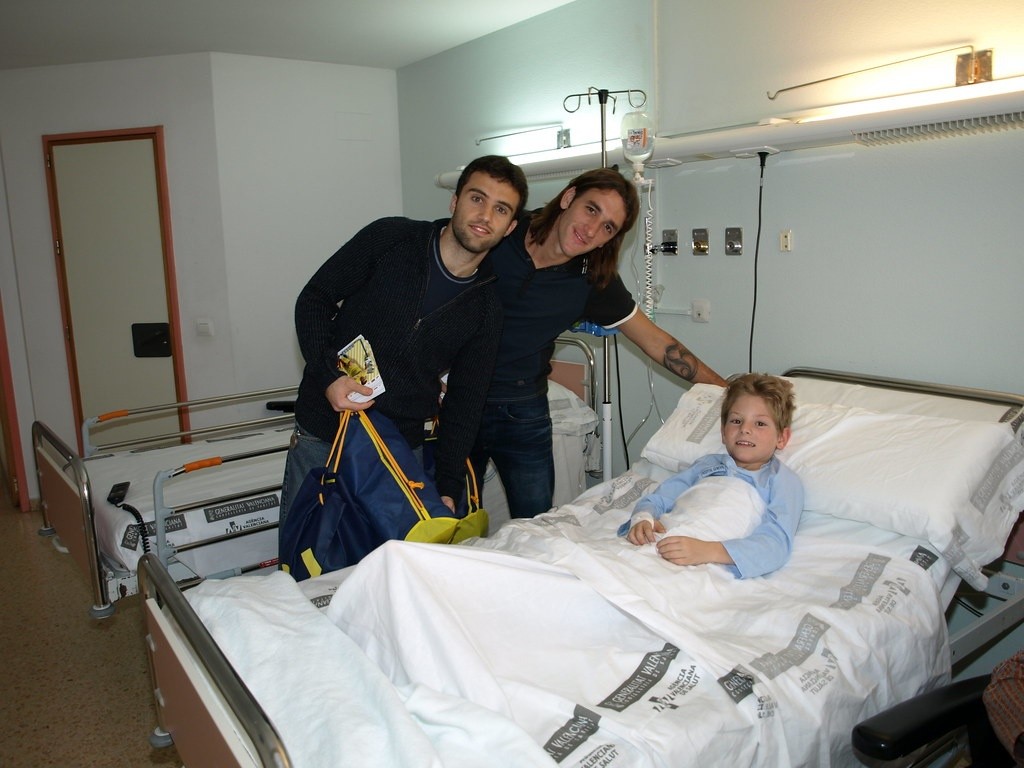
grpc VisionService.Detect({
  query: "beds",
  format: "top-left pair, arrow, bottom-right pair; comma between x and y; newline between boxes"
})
30,323 -> 1024,768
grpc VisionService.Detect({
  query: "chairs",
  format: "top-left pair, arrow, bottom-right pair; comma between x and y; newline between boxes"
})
851,673 -> 1024,768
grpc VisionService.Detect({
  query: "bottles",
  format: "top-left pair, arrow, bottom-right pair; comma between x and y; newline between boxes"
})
620,111 -> 657,172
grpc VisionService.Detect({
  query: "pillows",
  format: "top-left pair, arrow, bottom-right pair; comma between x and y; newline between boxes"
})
642,381 -> 1024,590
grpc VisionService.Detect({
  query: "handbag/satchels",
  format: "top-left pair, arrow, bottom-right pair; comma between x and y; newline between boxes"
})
276,400 -> 490,583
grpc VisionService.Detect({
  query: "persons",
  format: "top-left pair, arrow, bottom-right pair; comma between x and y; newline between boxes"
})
389,374 -> 805,655
428,167 -> 729,519
276,156 -> 529,576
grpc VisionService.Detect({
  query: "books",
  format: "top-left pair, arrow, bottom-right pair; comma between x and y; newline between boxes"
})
334,335 -> 386,403
